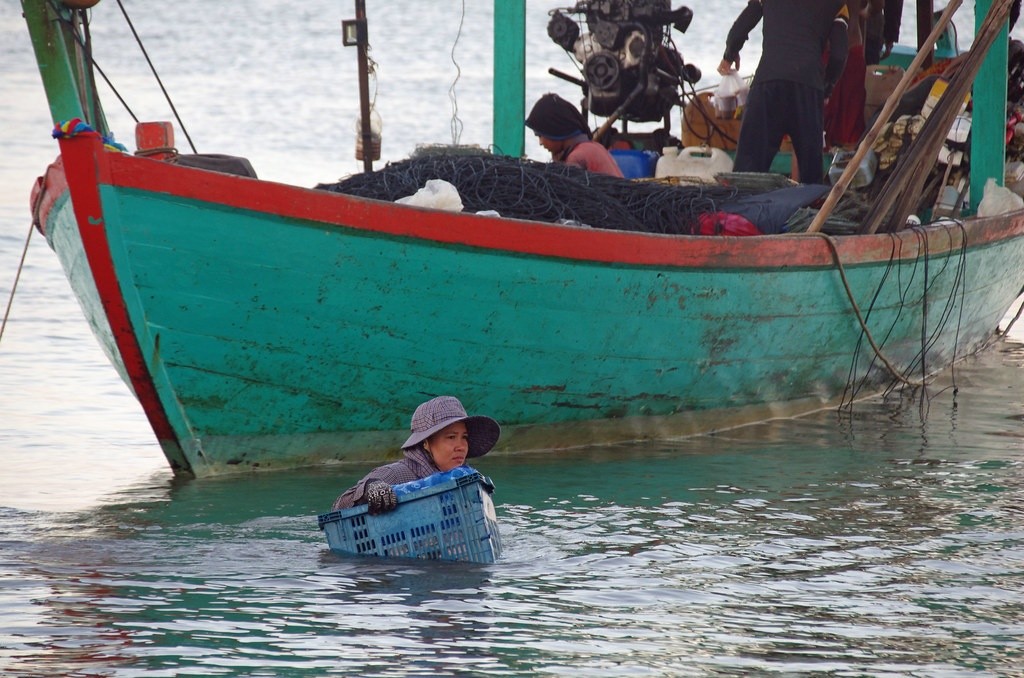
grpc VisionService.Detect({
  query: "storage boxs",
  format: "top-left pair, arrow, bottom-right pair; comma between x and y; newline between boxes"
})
318,466 -> 503,566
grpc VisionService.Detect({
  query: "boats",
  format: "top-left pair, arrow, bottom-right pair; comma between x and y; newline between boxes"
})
18,0 -> 1024,492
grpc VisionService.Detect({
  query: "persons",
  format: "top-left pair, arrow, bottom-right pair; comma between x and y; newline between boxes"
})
524,94 -> 623,179
717,0 -> 904,184
331,396 -> 501,515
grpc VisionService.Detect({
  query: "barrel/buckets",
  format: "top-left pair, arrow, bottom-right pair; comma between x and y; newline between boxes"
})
609,149 -> 651,181
655,146 -> 732,183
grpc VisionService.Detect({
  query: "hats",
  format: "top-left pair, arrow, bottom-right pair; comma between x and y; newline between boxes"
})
401,395 -> 500,458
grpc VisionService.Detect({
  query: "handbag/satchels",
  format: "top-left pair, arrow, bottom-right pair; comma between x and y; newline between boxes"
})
713,69 -> 750,119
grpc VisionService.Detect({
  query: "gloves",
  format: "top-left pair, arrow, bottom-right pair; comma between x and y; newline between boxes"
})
362,480 -> 398,516
